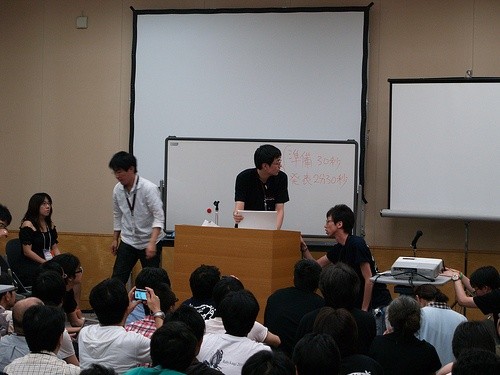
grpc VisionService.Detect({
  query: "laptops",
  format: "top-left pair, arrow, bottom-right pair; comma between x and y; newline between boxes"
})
237,210 -> 278,230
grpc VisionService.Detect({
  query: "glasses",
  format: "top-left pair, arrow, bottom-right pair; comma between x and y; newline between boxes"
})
472,286 -> 481,295
326,219 -> 333,224
73,267 -> 84,274
62,273 -> 67,278
272,160 -> 283,165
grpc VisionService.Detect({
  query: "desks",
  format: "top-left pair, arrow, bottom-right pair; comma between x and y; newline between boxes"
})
368,271 -> 451,298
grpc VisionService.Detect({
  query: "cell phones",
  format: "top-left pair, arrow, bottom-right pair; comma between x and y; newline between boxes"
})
134,288 -> 148,300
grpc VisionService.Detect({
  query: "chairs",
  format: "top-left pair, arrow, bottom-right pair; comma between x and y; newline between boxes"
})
6,239 -> 32,297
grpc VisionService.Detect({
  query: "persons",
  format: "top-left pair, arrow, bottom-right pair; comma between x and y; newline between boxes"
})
0,253 -> 500,375
108,152 -> 167,285
19,193 -> 84,325
233,144 -> 290,228
300,204 -> 393,336
0,203 -> 12,286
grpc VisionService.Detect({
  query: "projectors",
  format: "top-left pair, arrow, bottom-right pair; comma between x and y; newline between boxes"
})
390,256 -> 442,282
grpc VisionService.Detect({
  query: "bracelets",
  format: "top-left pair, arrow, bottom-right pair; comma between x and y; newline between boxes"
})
302,248 -> 308,252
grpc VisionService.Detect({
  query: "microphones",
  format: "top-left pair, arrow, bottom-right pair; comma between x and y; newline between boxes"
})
411,231 -> 423,247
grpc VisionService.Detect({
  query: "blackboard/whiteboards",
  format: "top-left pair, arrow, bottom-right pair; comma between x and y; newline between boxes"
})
158,136 -> 359,239
